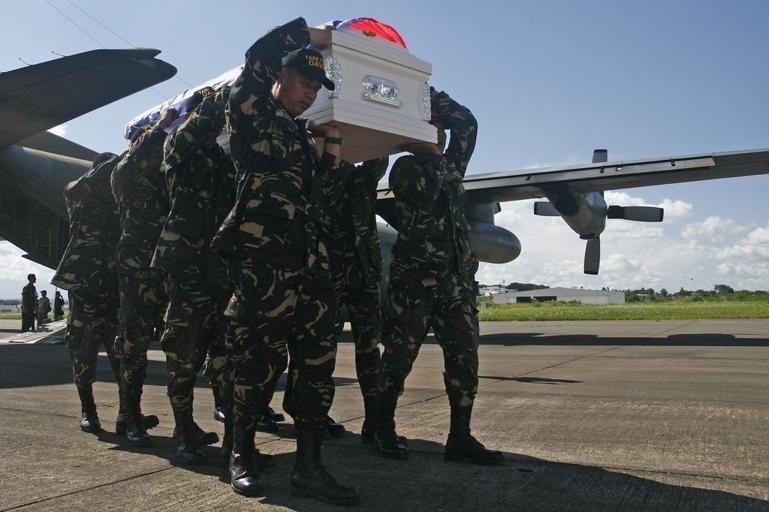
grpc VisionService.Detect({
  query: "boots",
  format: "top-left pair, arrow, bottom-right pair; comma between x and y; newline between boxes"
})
171,417 -> 220,445
229,425 -> 262,495
361,398 -> 409,448
288,440 -> 361,504
78,389 -> 101,432
126,393 -> 152,446
171,417 -> 208,466
268,406 -> 285,421
211,384 -> 227,421
117,393 -> 160,433
443,406 -> 503,463
255,406 -> 279,433
294,415 -> 346,436
370,395 -> 409,459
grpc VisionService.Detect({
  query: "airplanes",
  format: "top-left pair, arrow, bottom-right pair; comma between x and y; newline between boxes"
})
1,47 -> 769,348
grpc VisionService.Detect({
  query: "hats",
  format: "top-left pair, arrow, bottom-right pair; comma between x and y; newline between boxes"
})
131,123 -> 168,144
186,87 -> 216,110
91,152 -> 115,167
281,48 -> 335,91
